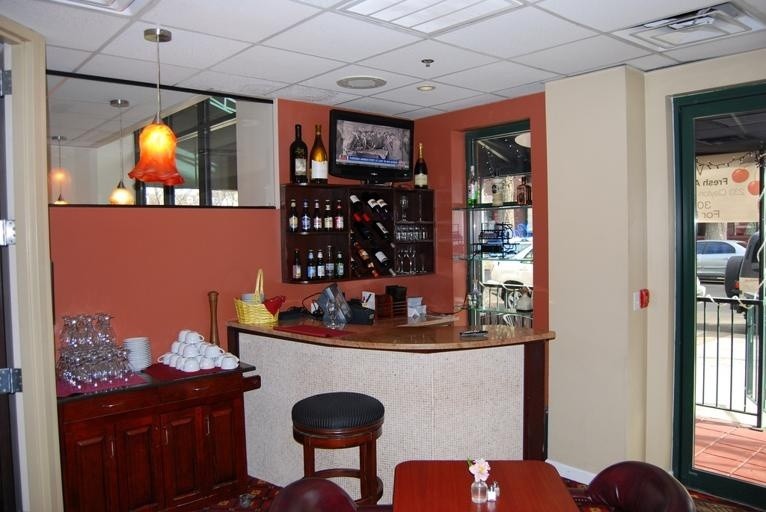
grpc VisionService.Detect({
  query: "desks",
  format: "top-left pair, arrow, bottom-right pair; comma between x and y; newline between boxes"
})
390,457 -> 579,512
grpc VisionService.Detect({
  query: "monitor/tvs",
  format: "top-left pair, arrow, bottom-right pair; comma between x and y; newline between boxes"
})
327,105 -> 415,188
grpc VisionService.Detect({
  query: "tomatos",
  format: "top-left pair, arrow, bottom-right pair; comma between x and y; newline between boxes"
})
747,180 -> 760,196
731,169 -> 748,183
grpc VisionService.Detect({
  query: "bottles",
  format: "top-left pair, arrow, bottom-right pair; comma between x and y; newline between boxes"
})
414,142 -> 428,189
305,250 -> 317,281
322,199 -> 333,232
493,481 -> 500,496
288,198 -> 299,233
325,245 -> 335,280
488,486 -> 497,501
334,251 -> 344,279
353,221 -> 380,248
517,177 -> 531,206
351,240 -> 379,278
372,221 -> 396,249
309,124 -> 329,184
311,199 -> 323,232
290,124 -> 308,186
300,198 -> 312,232
292,249 -> 302,282
349,191 -> 394,225
467,165 -> 479,209
368,241 -> 396,277
333,199 -> 344,232
316,249 -> 326,280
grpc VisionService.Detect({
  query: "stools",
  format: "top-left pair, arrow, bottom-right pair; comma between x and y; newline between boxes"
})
289,391 -> 385,512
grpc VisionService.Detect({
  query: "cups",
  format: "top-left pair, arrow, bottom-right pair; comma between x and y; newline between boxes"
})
200,343 -> 240,370
168,354 -> 179,368
171,340 -> 178,353
157,352 -> 173,365
396,224 -> 432,242
178,329 -> 191,342
183,357 -> 200,373
176,356 -> 187,371
178,342 -> 187,355
183,344 -> 201,357
185,331 -> 204,344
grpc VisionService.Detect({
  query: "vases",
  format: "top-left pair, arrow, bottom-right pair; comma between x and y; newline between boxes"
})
468,476 -> 487,504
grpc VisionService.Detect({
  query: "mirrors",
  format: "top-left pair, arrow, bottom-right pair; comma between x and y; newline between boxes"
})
46,70 -> 281,210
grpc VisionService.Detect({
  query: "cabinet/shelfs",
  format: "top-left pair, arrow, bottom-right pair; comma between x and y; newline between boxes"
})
165,372 -> 249,512
56,382 -> 165,512
282,185 -> 436,285
447,202 -> 535,319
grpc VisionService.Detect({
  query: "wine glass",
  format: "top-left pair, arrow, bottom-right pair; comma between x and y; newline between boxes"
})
55,312 -> 134,392
397,244 -> 433,277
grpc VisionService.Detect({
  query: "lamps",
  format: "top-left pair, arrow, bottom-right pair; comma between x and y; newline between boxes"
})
107,98 -> 138,206
126,27 -> 185,186
52,135 -> 71,205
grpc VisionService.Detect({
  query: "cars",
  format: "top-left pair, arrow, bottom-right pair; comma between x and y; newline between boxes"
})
487,242 -> 533,309
696,239 -> 747,283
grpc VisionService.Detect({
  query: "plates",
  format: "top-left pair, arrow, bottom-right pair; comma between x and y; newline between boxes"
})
123,336 -> 152,371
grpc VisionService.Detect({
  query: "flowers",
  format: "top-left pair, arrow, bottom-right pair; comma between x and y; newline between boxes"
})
467,457 -> 494,483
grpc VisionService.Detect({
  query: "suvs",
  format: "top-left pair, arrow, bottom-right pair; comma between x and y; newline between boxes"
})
726,232 -> 760,332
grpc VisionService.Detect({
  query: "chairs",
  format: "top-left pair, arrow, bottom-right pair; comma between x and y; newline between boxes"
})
566,462 -> 696,512
268,477 -> 356,511
